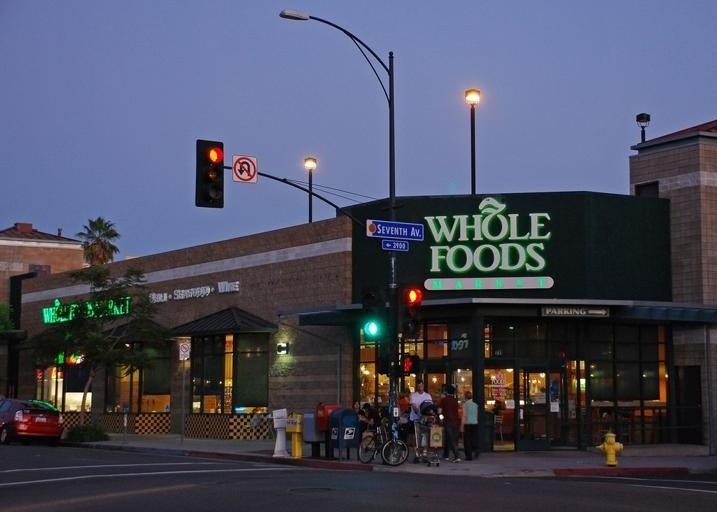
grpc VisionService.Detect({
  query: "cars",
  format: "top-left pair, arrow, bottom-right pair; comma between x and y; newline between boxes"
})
0,398 -> 65,445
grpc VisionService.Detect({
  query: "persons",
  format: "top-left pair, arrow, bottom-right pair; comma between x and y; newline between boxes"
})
408,381 -> 480,463
395,388 -> 412,424
350,400 -> 374,429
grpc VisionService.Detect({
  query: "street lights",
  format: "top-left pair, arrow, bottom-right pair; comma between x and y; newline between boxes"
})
277,5 -> 399,456
301,156 -> 318,223
462,86 -> 482,195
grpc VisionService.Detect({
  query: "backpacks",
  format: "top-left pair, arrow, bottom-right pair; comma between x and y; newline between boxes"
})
420,400 -> 437,416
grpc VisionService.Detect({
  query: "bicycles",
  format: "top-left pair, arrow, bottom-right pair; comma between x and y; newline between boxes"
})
356,416 -> 410,467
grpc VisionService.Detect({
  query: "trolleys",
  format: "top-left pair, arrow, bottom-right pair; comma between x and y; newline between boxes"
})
412,408 -> 444,468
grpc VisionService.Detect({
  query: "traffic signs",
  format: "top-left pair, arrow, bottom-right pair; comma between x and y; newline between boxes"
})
379,239 -> 408,252
364,218 -> 426,242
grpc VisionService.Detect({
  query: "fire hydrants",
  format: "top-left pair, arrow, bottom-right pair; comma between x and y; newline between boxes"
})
594,431 -> 625,468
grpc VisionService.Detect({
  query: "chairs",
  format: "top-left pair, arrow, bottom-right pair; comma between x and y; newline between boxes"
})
619,419 -> 632,445
599,416 -> 610,442
497,409 -> 513,436
494,415 -> 504,441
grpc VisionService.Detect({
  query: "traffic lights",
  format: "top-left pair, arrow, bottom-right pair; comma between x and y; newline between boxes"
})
362,290 -> 386,340
398,286 -> 424,341
398,354 -> 419,374
194,137 -> 224,209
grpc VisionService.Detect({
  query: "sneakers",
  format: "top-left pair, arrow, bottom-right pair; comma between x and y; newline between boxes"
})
442,456 -> 450,460
451,459 -> 461,463
